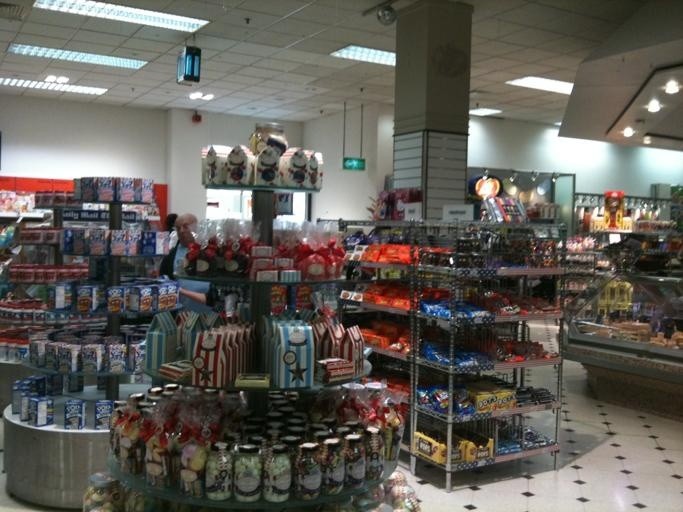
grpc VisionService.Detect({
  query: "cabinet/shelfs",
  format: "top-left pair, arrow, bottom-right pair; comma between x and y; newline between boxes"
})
555,227 -> 679,326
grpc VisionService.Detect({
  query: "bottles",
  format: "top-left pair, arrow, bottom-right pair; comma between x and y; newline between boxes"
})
576,195 -> 673,224
108,383 -> 404,505
